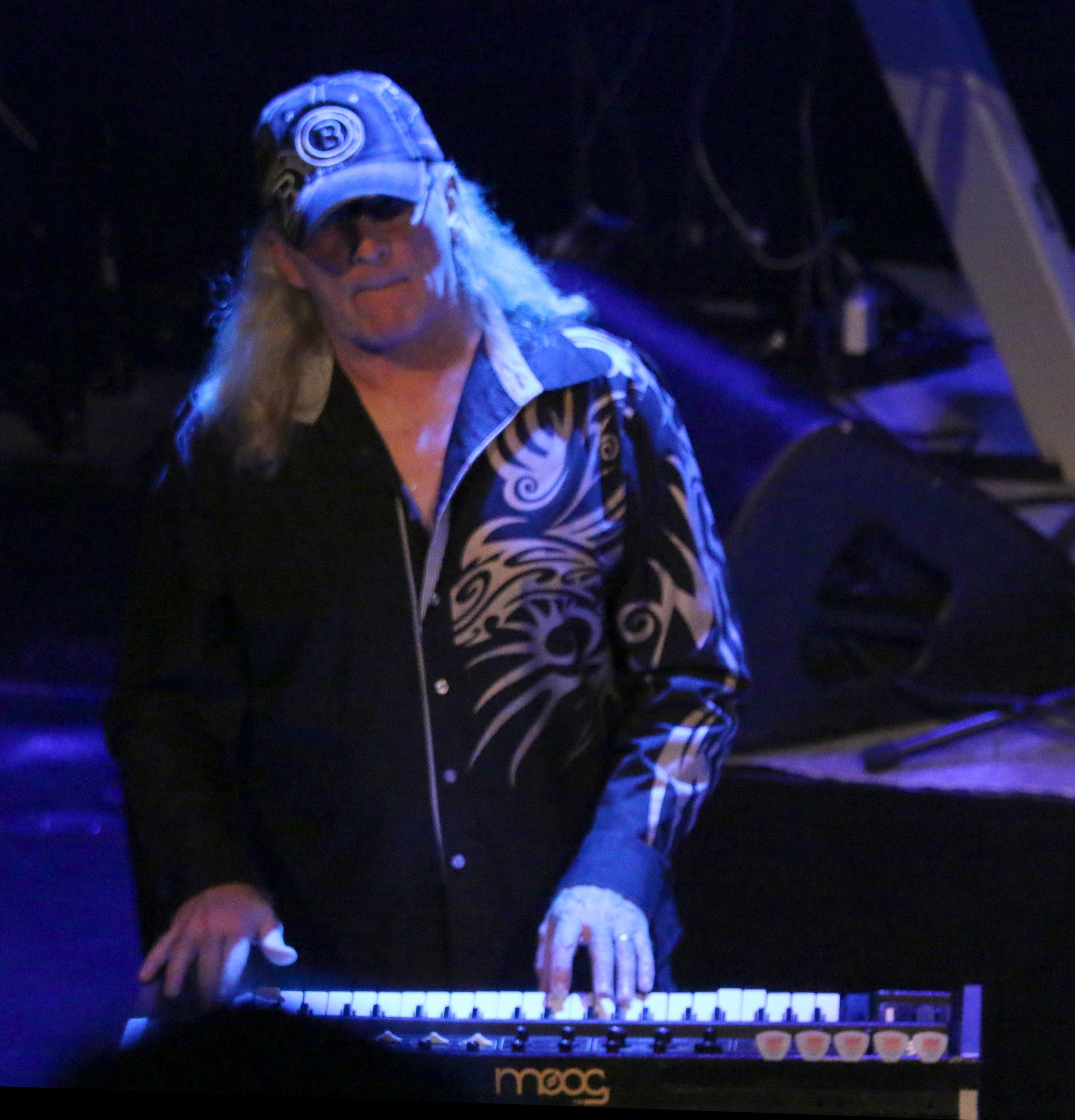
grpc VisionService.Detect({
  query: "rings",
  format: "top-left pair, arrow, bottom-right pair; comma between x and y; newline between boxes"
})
619,934 -> 632,941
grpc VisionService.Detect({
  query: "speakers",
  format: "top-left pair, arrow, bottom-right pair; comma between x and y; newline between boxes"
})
540,258 -> 1075,755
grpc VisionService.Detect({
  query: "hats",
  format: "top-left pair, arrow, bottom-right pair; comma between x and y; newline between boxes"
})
243,67 -> 447,251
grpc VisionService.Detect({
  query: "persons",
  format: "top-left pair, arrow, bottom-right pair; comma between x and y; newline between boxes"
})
100,71 -> 751,1023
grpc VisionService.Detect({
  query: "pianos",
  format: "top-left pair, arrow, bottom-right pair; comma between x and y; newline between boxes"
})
120,969 -> 984,1120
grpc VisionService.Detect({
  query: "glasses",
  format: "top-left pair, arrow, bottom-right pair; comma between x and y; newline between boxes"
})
303,194 -> 414,233
